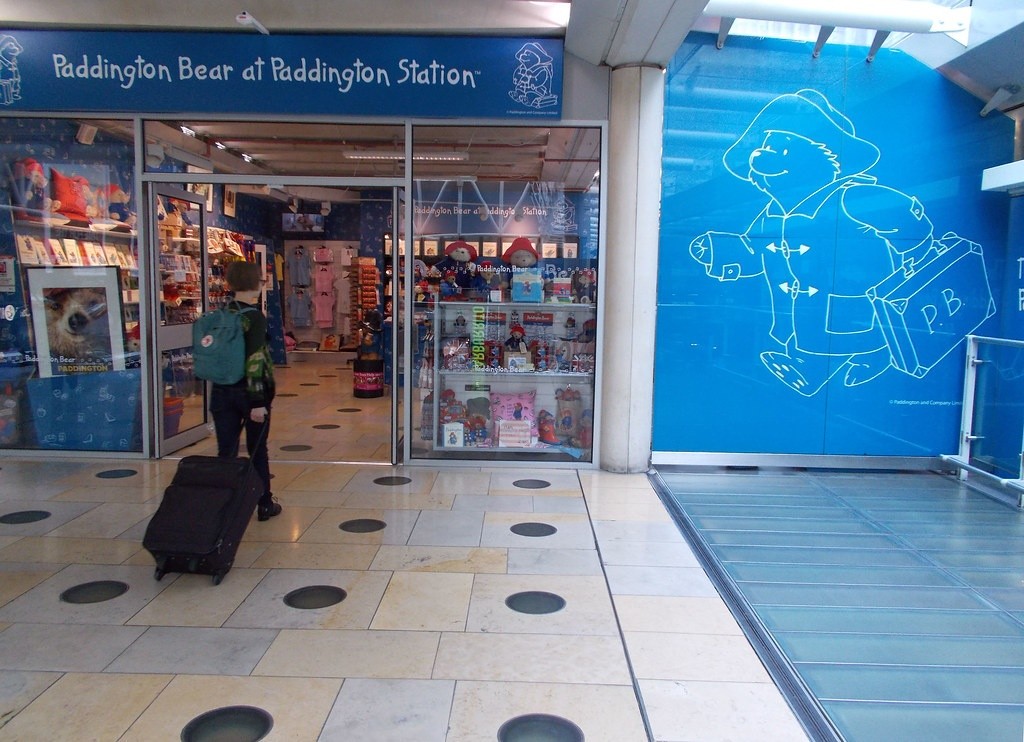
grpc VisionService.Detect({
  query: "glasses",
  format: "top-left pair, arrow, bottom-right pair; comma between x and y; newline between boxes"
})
259,280 -> 266,286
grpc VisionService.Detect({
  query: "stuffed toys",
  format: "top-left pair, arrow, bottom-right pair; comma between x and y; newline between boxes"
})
72,176 -> 137,226
440,390 -> 467,420
9,157 -> 61,217
177,203 -> 194,230
470,419 -> 491,446
415,237 -> 596,305
504,327 -> 528,351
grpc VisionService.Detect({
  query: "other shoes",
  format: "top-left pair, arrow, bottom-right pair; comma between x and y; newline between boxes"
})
258,502 -> 282,521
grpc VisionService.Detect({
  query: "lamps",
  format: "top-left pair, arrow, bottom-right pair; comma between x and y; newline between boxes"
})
288,199 -> 298,215
321,201 -> 331,216
342,140 -> 469,161
479,203 -> 488,221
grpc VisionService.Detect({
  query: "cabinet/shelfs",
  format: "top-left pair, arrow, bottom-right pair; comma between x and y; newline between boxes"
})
381,253 -> 435,389
432,291 -> 597,455
0,190 -> 200,389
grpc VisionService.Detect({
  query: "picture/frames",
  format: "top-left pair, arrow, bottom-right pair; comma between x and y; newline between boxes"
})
224,185 -> 236,219
24,265 -> 127,379
185,164 -> 213,212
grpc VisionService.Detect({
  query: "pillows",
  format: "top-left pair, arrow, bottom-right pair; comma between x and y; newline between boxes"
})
295,341 -> 320,352
339,342 -> 357,352
58,211 -> 91,227
318,332 -> 344,352
489,390 -> 541,440
51,168 -> 87,216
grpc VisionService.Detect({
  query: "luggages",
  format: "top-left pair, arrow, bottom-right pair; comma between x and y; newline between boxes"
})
142,411 -> 271,585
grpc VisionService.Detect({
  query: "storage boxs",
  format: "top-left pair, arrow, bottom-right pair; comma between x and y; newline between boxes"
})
163,397 -> 185,439
441,424 -> 464,447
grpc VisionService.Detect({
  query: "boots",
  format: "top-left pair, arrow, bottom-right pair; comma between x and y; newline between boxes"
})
542,423 -> 563,445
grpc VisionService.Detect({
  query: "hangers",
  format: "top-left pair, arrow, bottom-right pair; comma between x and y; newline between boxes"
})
343,273 -> 350,279
319,245 -> 326,249
321,292 -> 328,296
296,288 -> 304,294
295,246 -> 303,253
321,267 -> 327,271
347,246 -> 354,249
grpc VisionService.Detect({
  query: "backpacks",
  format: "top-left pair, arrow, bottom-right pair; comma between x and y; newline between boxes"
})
191,295 -> 261,385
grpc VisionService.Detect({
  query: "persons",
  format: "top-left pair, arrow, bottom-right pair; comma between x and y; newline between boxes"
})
538,410 -> 561,444
209,261 -> 282,522
571,409 -> 592,447
555,388 -> 580,400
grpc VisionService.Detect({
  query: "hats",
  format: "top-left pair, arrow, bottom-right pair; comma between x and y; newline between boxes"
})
358,311 -> 384,333
